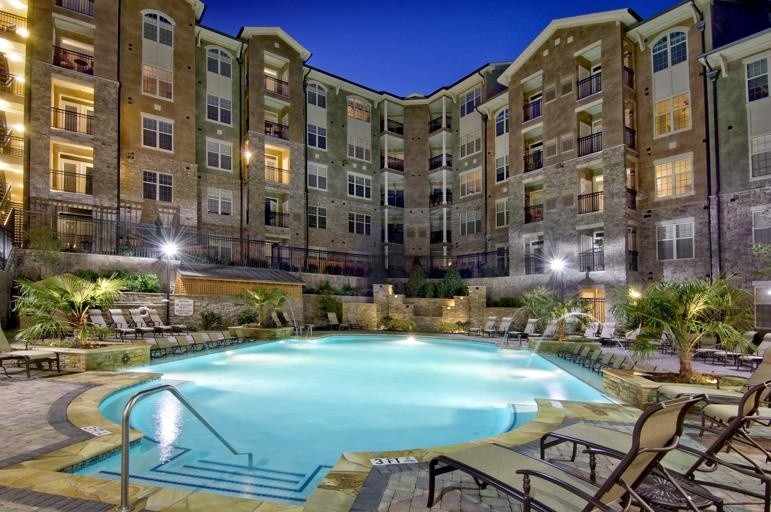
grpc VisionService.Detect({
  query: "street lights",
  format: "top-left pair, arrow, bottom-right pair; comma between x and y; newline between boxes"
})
159,241 -> 177,326
550,259 -> 566,312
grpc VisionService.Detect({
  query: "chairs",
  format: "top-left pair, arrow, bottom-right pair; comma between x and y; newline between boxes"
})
0,327 -> 61,379
268,309 -> 315,337
88,307 -> 257,361
326,311 -> 369,331
